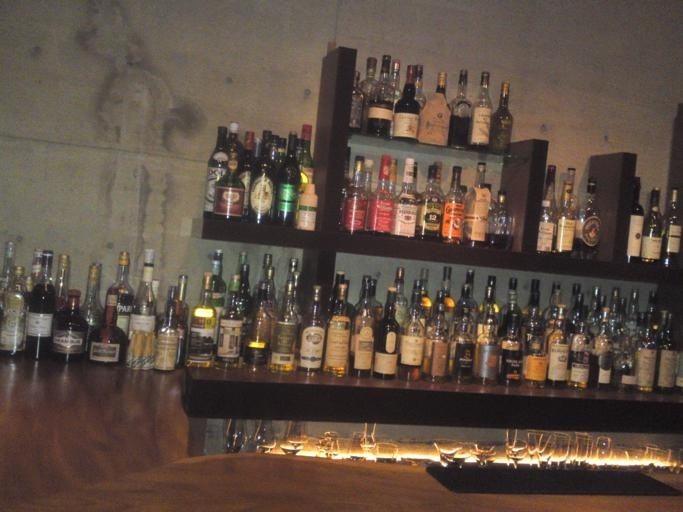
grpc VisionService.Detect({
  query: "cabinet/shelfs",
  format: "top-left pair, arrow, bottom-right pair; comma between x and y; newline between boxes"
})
180,47 -> 682,435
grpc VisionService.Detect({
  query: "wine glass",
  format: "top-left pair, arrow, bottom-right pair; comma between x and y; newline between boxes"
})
466,430 -> 683,471
319,424 -> 375,461
216,420 -> 306,458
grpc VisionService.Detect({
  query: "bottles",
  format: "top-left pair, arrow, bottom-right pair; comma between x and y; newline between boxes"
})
624,178 -> 682,271
337,55 -> 515,248
535,162 -> 603,256
202,120 -> 317,234
0,238 -> 682,392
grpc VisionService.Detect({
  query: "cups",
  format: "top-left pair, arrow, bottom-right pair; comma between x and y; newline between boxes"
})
433,439 -> 464,467
376,441 -> 399,463
